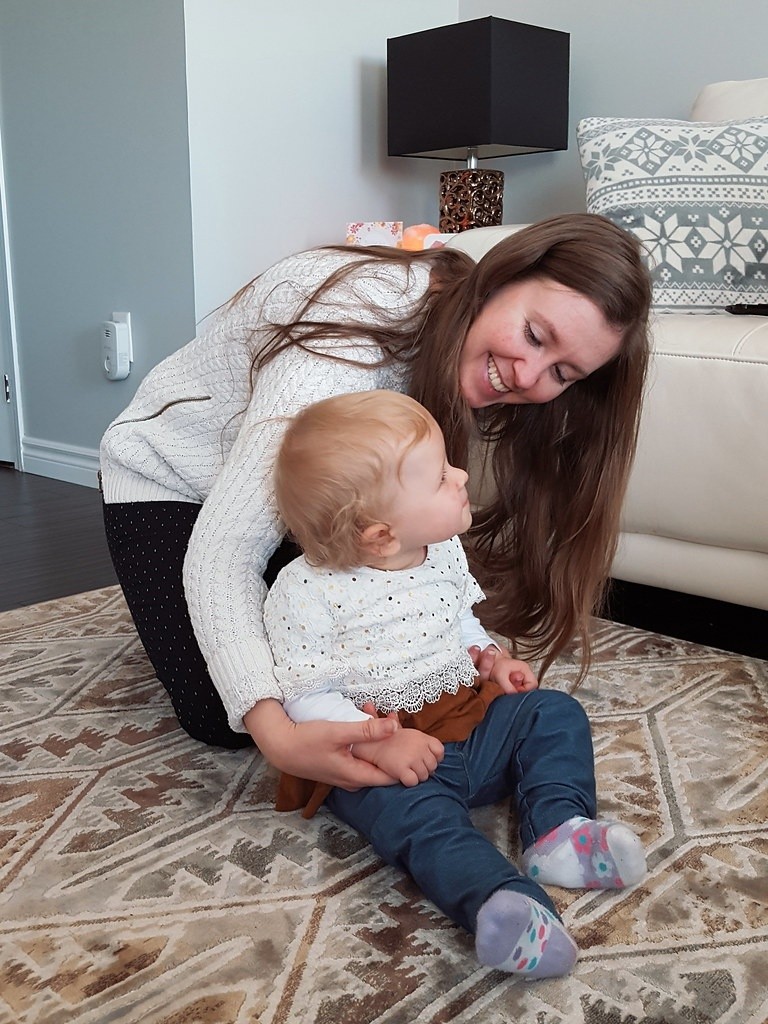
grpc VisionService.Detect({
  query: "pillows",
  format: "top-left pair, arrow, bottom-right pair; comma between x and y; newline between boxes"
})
574,115 -> 767,312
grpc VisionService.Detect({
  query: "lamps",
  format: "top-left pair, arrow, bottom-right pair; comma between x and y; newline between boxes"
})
382,14 -> 572,237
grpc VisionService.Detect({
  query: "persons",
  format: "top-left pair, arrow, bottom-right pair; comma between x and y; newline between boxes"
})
264,389 -> 649,982
97,210 -> 651,744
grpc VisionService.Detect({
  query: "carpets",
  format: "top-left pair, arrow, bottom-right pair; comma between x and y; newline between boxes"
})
0,573 -> 768,1023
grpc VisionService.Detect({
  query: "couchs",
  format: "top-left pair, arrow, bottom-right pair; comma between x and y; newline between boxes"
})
438,73 -> 768,613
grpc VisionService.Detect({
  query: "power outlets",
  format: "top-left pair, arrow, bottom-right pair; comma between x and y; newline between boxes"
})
111,309 -> 135,365
